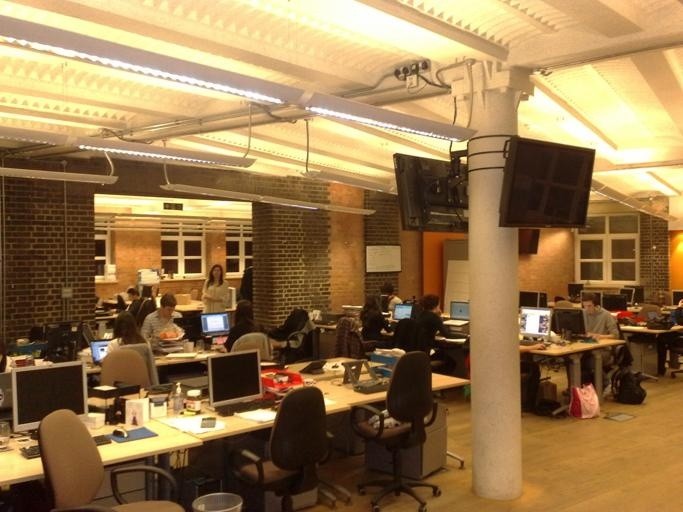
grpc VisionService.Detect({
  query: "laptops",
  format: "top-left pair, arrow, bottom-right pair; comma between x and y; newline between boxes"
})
393,304 -> 413,320
0,372 -> 12,421
89,338 -> 113,367
442,301 -> 470,327
173,317 -> 201,347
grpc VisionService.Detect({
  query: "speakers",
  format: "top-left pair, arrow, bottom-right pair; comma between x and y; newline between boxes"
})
518,228 -> 540,253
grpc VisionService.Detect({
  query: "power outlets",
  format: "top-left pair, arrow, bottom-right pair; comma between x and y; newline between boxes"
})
394,60 -> 430,88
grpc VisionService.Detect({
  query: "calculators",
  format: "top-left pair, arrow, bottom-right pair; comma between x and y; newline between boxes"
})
200,417 -> 216,428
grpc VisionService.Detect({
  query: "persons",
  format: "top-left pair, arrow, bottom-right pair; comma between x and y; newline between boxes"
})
201,263 -> 230,314
219,298 -> 257,353
379,282 -> 403,313
414,293 -> 457,375
358,296 -> 392,348
519,343 -> 547,412
107,288 -> 183,354
581,292 -> 620,375
670,298 -> 683,369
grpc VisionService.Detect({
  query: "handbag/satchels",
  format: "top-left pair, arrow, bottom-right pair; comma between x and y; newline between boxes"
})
646,316 -> 674,330
568,382 -> 600,419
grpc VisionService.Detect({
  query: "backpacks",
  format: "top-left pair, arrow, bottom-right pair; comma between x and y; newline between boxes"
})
611,365 -> 645,405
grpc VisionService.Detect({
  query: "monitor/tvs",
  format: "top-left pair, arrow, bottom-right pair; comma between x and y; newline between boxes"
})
580,291 -> 603,309
624,285 -> 644,303
11,360 -> 89,440
518,291 -> 539,314
568,284 -> 584,303
540,293 -> 548,307
519,306 -> 554,342
497,135 -> 596,228
393,153 -> 468,233
552,309 -> 587,346
672,290 -> 683,310
207,348 -> 263,413
602,294 -> 627,310
200,312 -> 231,337
619,288 -> 636,307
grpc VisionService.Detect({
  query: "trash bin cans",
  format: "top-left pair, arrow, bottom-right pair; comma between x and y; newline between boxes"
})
191,493 -> 243,512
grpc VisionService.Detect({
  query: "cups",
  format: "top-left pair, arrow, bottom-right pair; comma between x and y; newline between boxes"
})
0,421 -> 10,452
187,342 -> 195,352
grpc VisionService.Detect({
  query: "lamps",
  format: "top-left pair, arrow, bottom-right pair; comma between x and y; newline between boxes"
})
0,152 -> 120,186
590,179 -> 678,223
69,105 -> 257,168
301,120 -> 398,197
160,164 -> 376,216
304,92 -> 477,142
0,15 -> 305,105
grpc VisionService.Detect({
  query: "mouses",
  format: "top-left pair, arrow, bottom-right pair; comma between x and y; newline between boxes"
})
112,428 -> 129,438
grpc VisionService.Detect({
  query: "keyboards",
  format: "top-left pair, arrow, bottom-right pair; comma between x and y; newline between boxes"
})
572,335 -> 594,340
520,340 -> 544,346
19,435 -> 112,459
214,398 -> 275,417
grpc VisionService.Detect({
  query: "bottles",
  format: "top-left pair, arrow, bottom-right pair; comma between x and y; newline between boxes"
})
196,340 -> 204,353
185,388 -> 202,415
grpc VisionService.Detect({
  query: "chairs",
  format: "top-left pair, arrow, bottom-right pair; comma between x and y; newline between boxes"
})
0,298 -> 471,510
519,299 -> 682,421
